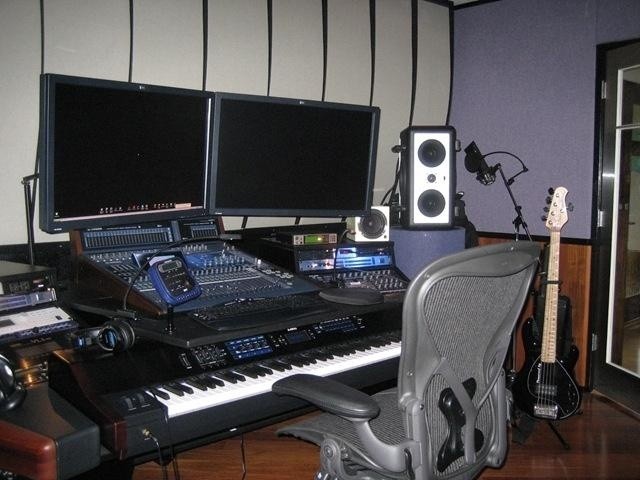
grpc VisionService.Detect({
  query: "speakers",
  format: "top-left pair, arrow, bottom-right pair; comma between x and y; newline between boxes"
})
400,125 -> 456,231
347,205 -> 391,243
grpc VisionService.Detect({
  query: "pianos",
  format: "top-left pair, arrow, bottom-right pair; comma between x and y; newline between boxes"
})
48,301 -> 402,461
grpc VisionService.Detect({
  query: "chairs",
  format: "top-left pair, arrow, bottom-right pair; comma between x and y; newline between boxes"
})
271,240 -> 541,480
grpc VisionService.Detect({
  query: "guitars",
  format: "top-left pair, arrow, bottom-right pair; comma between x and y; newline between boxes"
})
511,187 -> 582,420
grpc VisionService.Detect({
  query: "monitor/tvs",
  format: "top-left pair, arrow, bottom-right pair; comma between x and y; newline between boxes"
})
40,73 -> 214,235
206,91 -> 381,219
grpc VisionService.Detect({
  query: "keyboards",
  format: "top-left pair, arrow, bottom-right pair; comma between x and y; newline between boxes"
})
189,291 -> 337,331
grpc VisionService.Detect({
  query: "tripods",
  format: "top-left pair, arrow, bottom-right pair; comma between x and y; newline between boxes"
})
493,160 -> 570,452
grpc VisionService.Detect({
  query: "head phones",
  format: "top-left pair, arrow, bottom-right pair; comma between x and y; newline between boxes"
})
68,321 -> 135,351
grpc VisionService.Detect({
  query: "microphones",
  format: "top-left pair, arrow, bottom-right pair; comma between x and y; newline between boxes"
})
463,140 -> 497,186
